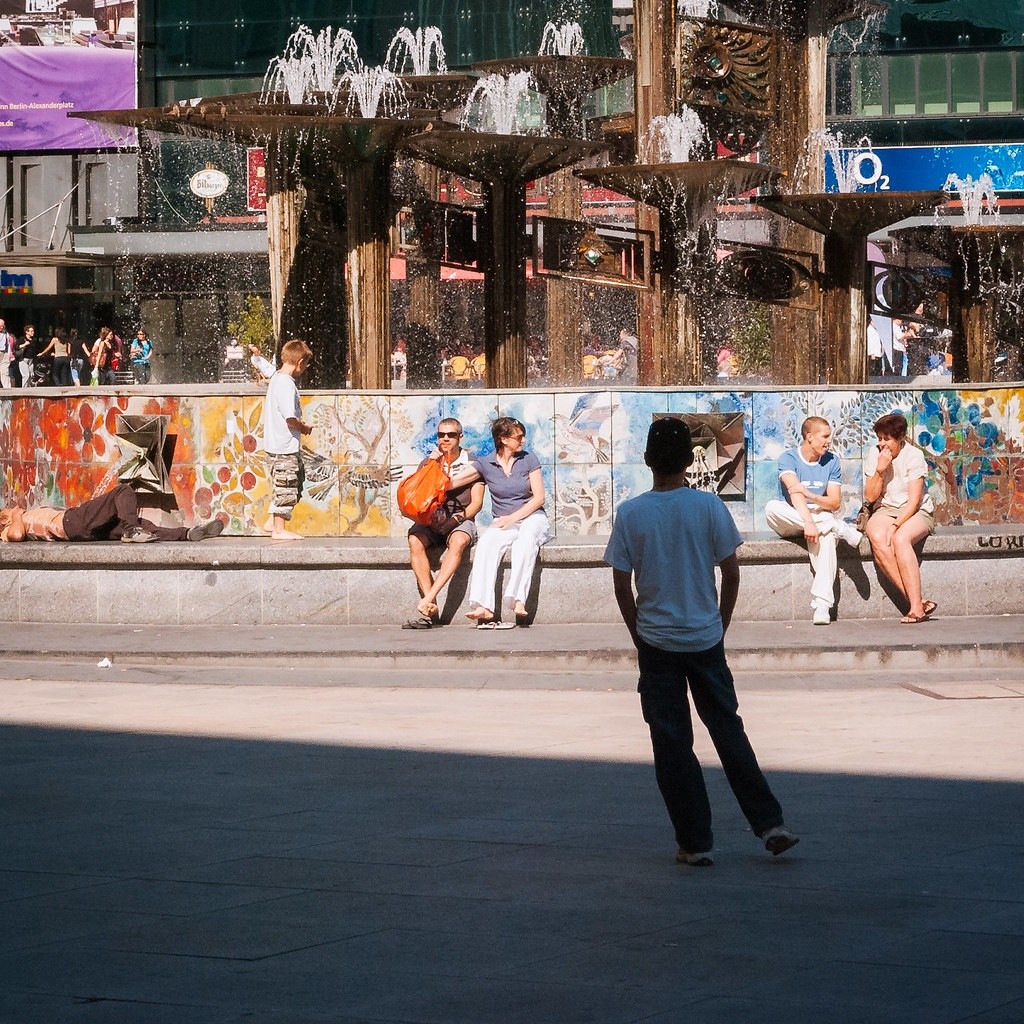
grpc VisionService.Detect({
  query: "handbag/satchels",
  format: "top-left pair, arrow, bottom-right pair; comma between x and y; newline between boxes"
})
89,366 -> 99,386
856,501 -> 874,532
88,351 -> 107,368
12,348 -> 25,362
396,443 -> 459,527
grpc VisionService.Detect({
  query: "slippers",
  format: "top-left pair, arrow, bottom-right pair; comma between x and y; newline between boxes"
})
416,618 -> 442,629
900,613 -> 929,624
922,599 -> 938,615
494,620 -> 516,629
476,621 -> 495,629
401,619 -> 419,629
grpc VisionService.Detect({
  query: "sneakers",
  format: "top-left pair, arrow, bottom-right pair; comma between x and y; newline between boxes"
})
187,519 -> 225,541
676,847 -> 714,866
120,525 -> 161,543
832,519 -> 863,548
760,824 -> 801,856
813,608 -> 831,625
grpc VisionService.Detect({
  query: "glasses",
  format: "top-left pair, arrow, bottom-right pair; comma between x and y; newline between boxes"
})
136,334 -> 144,335
505,435 -> 525,442
437,432 -> 457,438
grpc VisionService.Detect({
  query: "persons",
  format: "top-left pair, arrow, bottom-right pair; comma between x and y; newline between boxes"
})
438,417 -> 553,620
36,327 -> 69,386
0,319 -> 16,388
715,343 -> 736,377
0,484 -> 224,543
892,319 -> 933,376
867,319 -> 883,376
393,338 -> 407,388
250,344 -> 276,378
15,325 -> 36,387
407,417 -> 486,615
582,326 -> 638,379
130,331 -> 152,384
864,414 -> 937,623
766,417 -> 863,625
67,328 -> 92,386
603,418 -> 800,866
264,340 -> 314,540
92,326 -> 123,383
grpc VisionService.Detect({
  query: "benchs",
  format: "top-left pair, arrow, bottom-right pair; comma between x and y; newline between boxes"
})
0,523 -> 1024,624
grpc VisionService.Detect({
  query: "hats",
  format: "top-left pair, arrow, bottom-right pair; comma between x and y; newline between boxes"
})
648,417 -> 691,447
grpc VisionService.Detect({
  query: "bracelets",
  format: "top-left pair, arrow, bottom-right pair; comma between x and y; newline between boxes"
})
876,468 -> 884,474
892,524 -> 899,527
